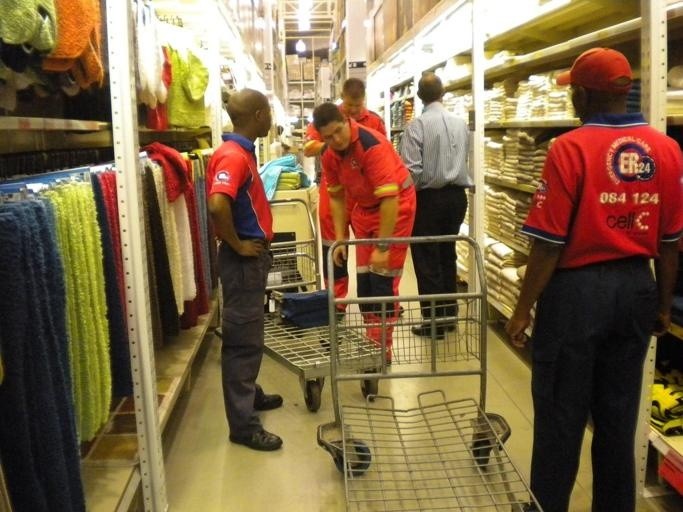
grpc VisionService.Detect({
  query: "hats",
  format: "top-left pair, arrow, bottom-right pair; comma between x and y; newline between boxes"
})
557,47 -> 633,94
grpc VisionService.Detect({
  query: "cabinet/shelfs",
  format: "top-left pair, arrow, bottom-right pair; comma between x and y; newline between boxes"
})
473,0 -> 651,428
636,0 -> 682,511
1,1 -> 225,512
406,0 -> 471,310
226,0 -> 407,172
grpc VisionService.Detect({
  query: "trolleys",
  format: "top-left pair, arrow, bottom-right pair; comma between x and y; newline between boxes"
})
211,197 -> 389,411
315,234 -> 545,510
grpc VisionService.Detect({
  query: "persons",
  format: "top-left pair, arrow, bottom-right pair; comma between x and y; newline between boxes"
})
303,79 -> 403,319
204,90 -> 282,450
505,47 -> 683,512
313,103 -> 417,365
401,74 -> 474,340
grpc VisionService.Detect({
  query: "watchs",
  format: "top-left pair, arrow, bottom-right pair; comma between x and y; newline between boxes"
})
377,243 -> 390,252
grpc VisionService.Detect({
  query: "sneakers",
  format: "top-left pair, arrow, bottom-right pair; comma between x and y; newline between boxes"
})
254,395 -> 282,411
229,430 -> 282,451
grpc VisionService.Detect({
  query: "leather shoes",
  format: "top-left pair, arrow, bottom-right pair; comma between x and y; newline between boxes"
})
412,323 -> 457,339
